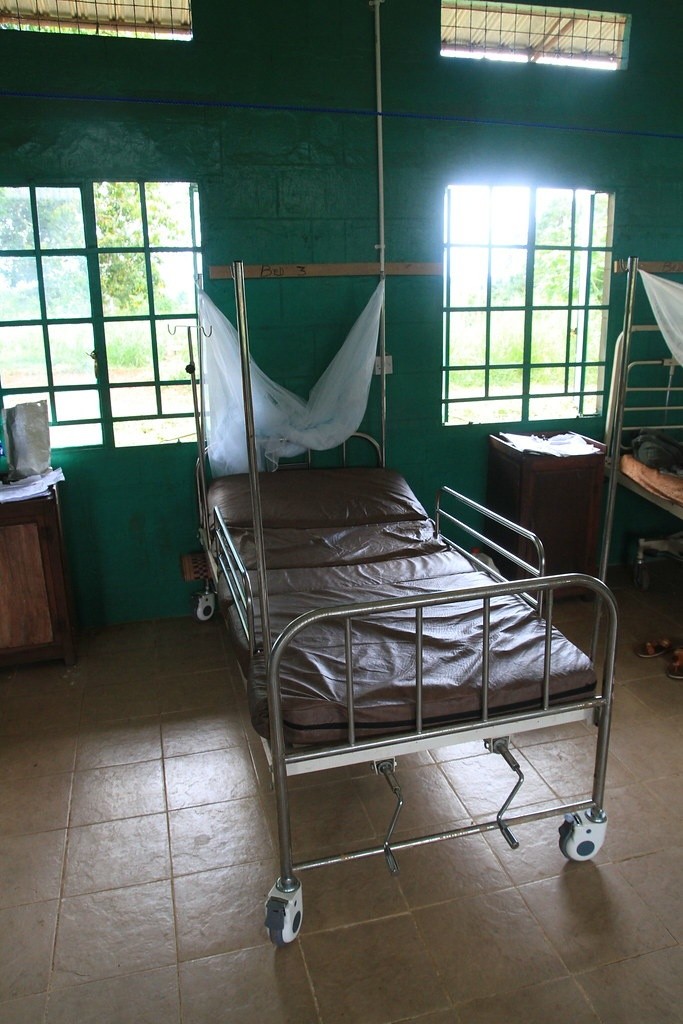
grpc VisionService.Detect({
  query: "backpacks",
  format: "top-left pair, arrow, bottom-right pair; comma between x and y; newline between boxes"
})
620,428 -> 683,477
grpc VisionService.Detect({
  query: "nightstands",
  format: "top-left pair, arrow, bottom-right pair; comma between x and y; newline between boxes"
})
487,431 -> 607,601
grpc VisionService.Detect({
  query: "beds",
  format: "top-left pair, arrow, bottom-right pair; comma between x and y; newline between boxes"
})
602,326 -> 683,586
195,431 -> 619,946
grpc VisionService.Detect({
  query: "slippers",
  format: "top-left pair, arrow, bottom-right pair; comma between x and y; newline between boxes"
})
667,647 -> 683,679
635,635 -> 683,657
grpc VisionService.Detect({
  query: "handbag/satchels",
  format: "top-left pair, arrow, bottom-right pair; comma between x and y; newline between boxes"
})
2,400 -> 51,480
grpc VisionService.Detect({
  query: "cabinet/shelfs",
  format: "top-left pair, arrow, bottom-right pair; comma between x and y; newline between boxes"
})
0,466 -> 76,666
